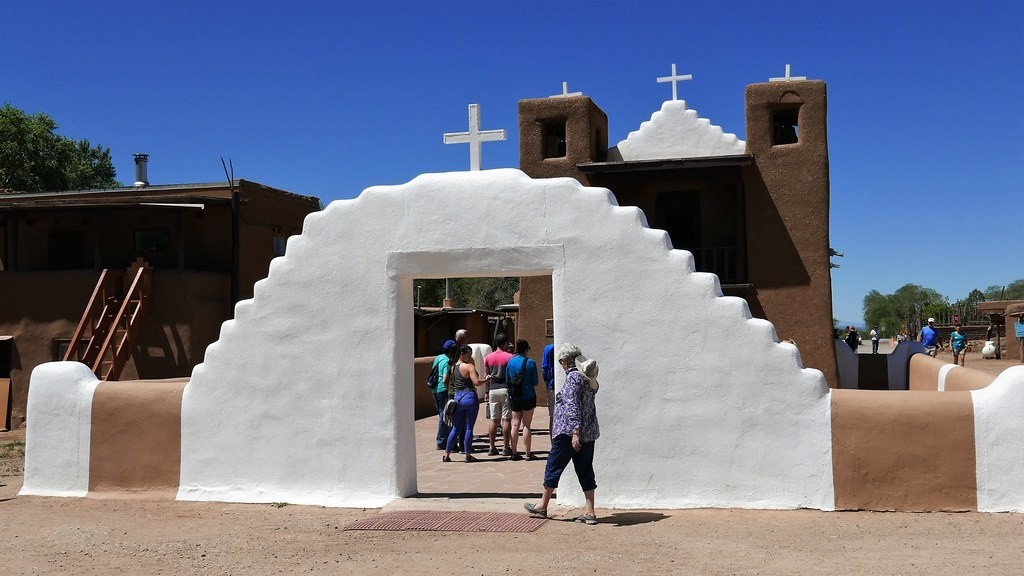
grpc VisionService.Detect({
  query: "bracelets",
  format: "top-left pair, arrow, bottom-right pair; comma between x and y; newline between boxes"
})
573,430 -> 579,434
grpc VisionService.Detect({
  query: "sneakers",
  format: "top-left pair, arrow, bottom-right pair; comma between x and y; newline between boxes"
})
523,503 -> 547,518
573,514 -> 597,524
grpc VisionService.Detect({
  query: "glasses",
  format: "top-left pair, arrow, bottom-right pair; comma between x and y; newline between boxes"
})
558,359 -> 563,365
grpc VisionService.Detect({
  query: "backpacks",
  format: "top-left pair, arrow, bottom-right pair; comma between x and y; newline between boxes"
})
426,356 -> 446,389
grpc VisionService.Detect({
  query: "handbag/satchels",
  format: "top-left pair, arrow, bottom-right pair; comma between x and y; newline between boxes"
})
877,337 -> 880,340
506,372 -> 524,399
446,402 -> 457,416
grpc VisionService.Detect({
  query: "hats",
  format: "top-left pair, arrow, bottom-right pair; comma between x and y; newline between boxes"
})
443,340 -> 455,349
575,355 -> 599,389
874,326 -> 877,329
927,318 -> 934,322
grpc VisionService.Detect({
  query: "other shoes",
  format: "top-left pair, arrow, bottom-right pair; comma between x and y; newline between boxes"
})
442,456 -> 451,462
465,456 -> 478,463
488,448 -> 499,456
437,445 -> 442,450
502,448 -> 513,456
497,425 -> 502,431
510,454 -> 523,461
525,453 -> 539,461
450,446 -> 458,452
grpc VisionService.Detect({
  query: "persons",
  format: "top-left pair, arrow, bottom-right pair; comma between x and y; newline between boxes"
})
541,342 -> 555,445
441,345 -> 493,462
949,324 -> 969,368
484,333 -> 516,455
869,326 -> 880,354
430,340 -> 456,449
455,329 -> 470,346
916,318 -> 944,358
523,343 -> 600,524
845,325 -> 859,353
505,339 -> 539,461
